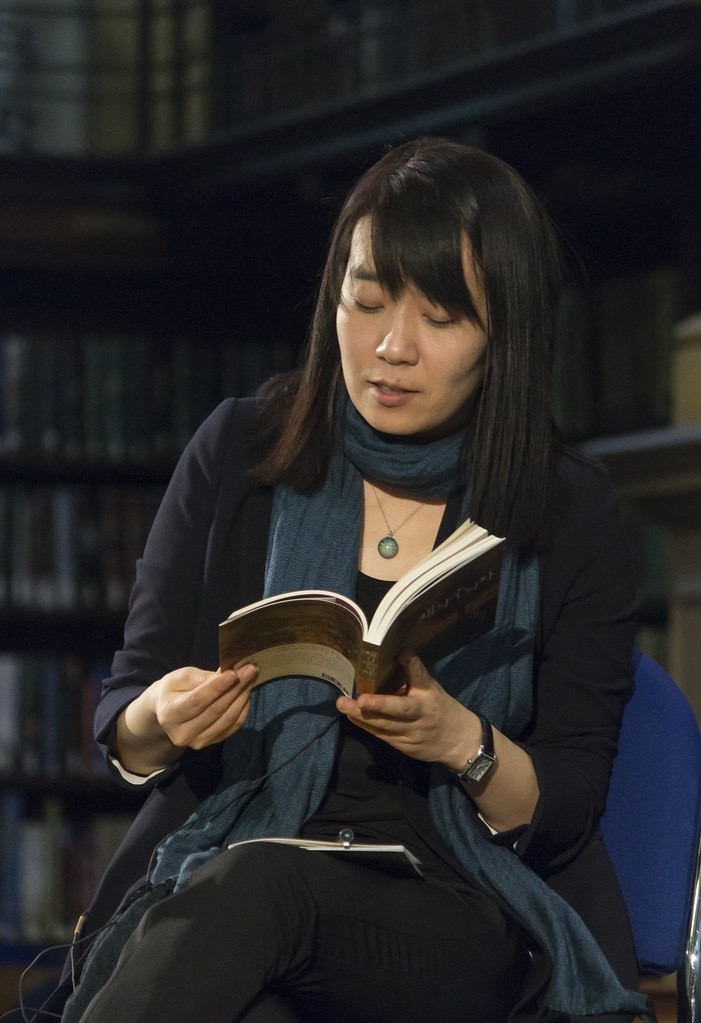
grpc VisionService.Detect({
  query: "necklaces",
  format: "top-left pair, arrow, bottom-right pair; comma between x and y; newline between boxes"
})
371,482 -> 427,558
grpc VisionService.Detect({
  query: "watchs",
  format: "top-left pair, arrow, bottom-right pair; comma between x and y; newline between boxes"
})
449,712 -> 495,785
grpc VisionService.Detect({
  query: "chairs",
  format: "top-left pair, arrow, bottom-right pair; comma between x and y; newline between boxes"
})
594,652 -> 700,1020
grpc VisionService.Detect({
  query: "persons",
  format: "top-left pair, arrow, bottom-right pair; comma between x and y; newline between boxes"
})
57,138 -> 655,1023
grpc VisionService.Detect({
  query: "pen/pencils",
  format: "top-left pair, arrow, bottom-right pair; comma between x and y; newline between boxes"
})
336,828 -> 353,845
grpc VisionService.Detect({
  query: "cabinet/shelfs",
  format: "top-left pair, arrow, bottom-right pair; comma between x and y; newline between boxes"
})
0,438 -> 178,806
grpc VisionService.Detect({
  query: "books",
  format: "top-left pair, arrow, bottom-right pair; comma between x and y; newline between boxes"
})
219,518 -> 506,700
0,0 -> 701,979
229,837 -> 423,877
